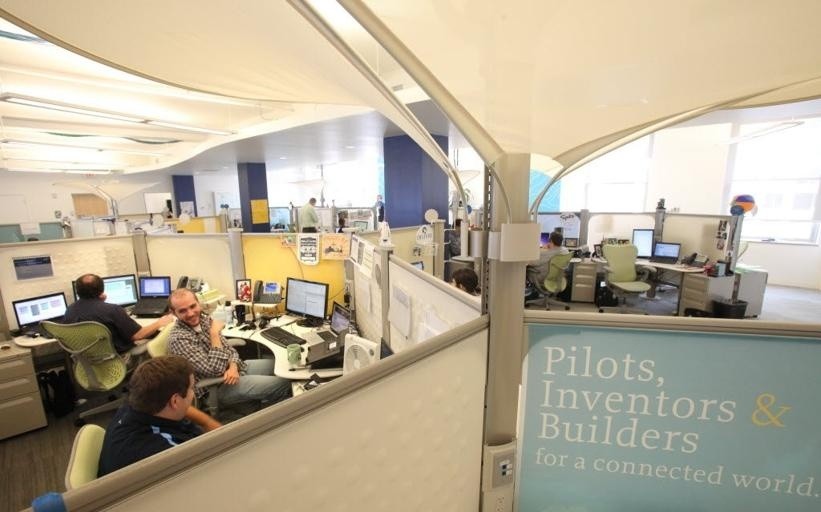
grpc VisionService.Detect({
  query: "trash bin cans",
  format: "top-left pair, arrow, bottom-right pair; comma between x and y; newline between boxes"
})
711,297 -> 748,318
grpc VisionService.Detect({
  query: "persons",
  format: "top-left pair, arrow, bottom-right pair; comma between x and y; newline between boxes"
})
337,217 -> 349,233
450,268 -> 480,299
63,274 -> 173,378
97,355 -> 223,481
374,195 -> 386,221
525,230 -> 569,285
166,287 -> 292,410
302,198 -> 319,233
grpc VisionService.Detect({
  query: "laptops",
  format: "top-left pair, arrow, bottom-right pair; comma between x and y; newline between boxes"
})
317,300 -> 352,341
650,242 -> 681,264
12,292 -> 68,336
135,276 -> 171,317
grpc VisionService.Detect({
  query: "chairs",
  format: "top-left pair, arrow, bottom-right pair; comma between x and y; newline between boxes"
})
598,243 -> 652,315
38,319 -> 134,424
63,423 -> 108,496
146,324 -> 175,360
523,250 -> 575,310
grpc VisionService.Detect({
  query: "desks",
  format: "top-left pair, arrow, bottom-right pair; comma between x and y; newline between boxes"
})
11,272 -> 382,383
591,254 -> 707,273
570,256 -> 582,264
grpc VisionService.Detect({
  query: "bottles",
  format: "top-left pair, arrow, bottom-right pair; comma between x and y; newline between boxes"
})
223,301 -> 233,322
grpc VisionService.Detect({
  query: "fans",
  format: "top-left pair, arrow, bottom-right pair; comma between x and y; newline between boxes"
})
342,333 -> 380,381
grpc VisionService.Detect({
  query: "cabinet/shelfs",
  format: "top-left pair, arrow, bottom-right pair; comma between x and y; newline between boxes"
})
570,262 -> 598,303
0,339 -> 49,438
676,273 -> 735,317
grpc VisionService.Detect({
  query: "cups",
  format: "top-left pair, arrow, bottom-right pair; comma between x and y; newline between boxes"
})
287,344 -> 301,364
232,304 -> 245,322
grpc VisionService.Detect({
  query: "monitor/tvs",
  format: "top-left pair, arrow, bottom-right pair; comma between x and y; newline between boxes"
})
284,278 -> 329,327
72,274 -> 138,308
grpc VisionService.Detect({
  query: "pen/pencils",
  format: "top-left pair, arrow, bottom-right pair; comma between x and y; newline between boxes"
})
290,365 -> 309,371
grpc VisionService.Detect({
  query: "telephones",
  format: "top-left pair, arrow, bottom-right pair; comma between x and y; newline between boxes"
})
252,280 -> 283,304
176,275 -> 202,292
687,252 -> 708,268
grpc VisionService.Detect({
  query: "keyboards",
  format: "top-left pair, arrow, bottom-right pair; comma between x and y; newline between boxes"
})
260,327 -> 306,350
